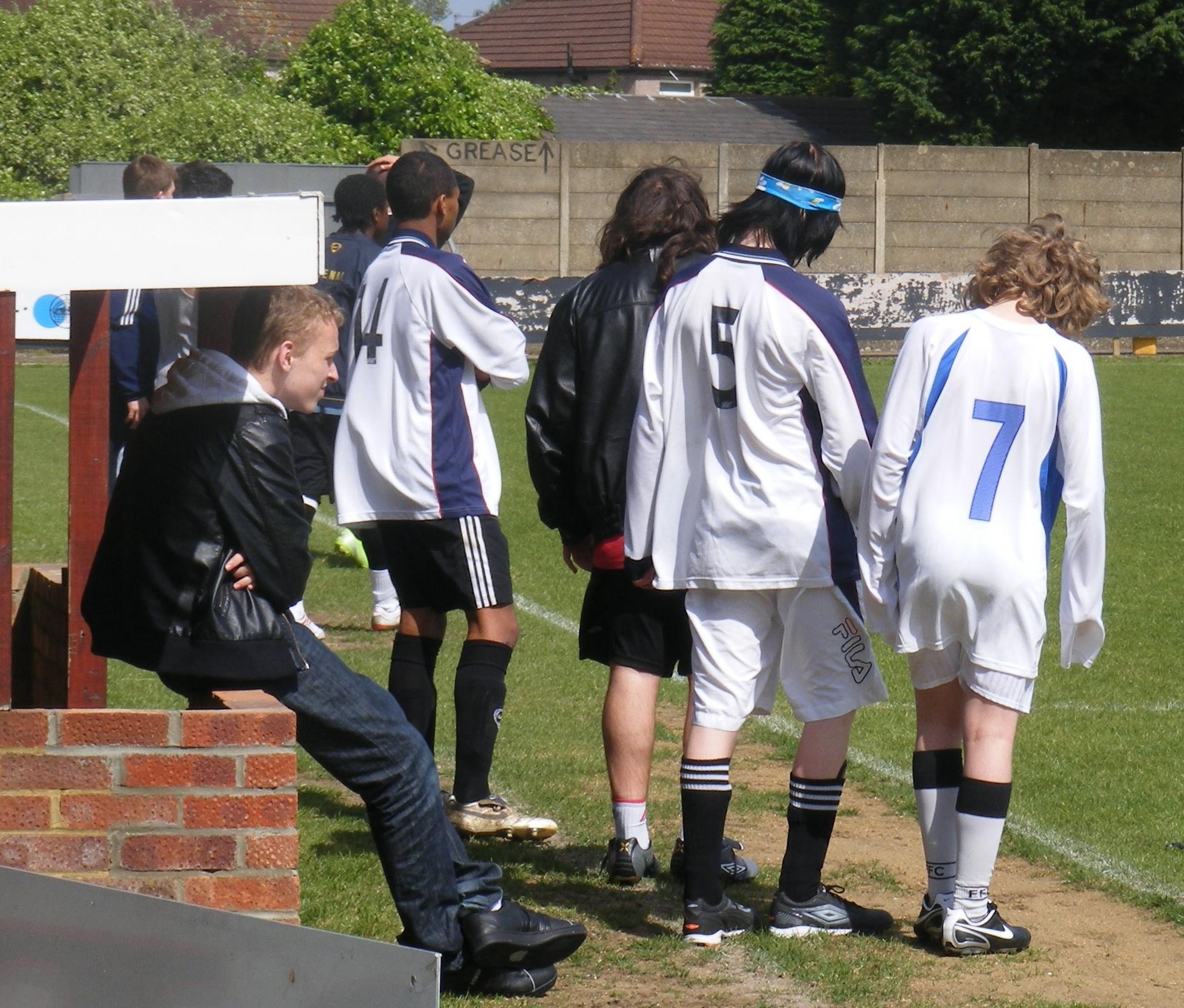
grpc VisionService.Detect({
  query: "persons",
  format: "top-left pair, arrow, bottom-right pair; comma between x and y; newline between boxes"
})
83,285 -> 587,999
623,141 -> 895,938
526,157 -> 755,885
109,152 -> 561,840
860,217 -> 1106,957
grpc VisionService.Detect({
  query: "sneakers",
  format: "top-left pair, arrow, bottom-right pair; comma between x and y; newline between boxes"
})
598,836 -> 661,884
669,836 -> 758,884
446,796 -> 557,841
768,882 -> 894,939
437,789 -> 457,816
371,603 -> 402,629
941,899 -> 1032,959
682,895 -> 756,947
461,898 -> 588,968
299,614 -> 326,640
441,956 -> 558,996
914,888 -> 955,940
